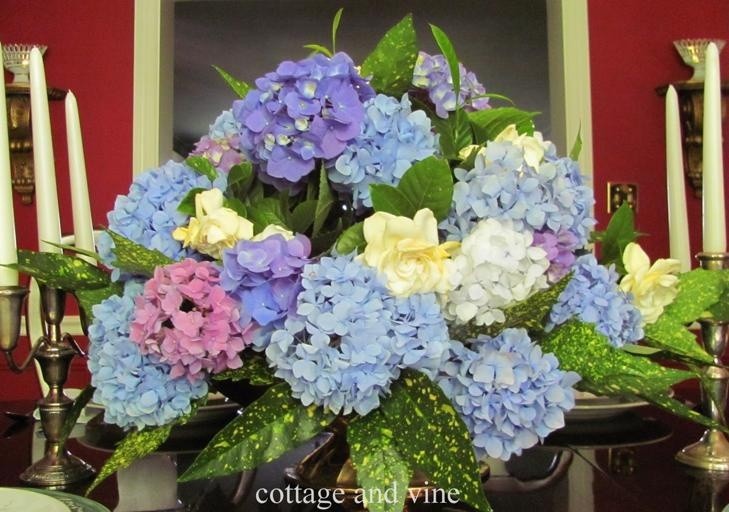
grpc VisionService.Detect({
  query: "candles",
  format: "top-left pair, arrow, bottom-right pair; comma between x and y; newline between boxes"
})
0,47 -> 95,290
664,40 -> 726,276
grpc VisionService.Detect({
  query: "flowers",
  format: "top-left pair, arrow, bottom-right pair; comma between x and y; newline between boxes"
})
0,2 -> 726,512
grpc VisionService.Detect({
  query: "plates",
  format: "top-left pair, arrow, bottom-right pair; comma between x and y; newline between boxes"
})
546,386 -> 675,421
